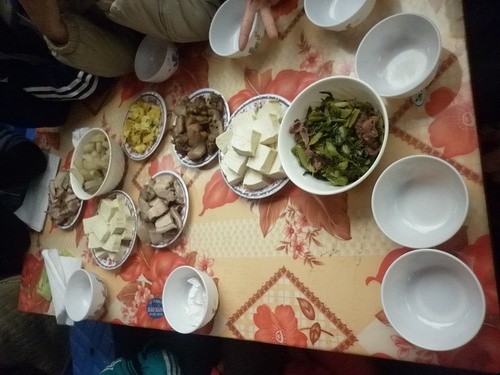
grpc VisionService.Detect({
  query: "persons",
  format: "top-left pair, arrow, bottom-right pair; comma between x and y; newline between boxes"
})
0,0 -> 286,375
100,328 -> 212,375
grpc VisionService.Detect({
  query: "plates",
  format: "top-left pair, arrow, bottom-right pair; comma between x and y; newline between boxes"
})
218,94 -> 291,199
121,92 -> 167,161
139,169 -> 189,248
172,88 -> 230,168
91,190 -> 137,270
59,200 -> 83,229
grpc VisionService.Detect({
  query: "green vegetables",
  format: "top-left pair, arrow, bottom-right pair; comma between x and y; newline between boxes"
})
288,91 -> 384,186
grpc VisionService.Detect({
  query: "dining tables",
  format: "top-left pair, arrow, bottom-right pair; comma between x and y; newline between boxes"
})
0,0 -> 500,375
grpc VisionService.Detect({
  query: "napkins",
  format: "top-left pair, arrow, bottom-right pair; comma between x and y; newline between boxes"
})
12,152 -> 61,232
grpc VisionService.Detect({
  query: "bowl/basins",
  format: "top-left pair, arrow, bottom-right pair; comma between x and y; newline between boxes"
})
70,127 -> 126,200
355,13 -> 442,99
304,0 -> 376,32
209,0 -> 266,59
64,269 -> 107,322
134,35 -> 179,82
278,76 -> 389,195
381,249 -> 487,351
162,265 -> 219,334
371,154 -> 470,249
